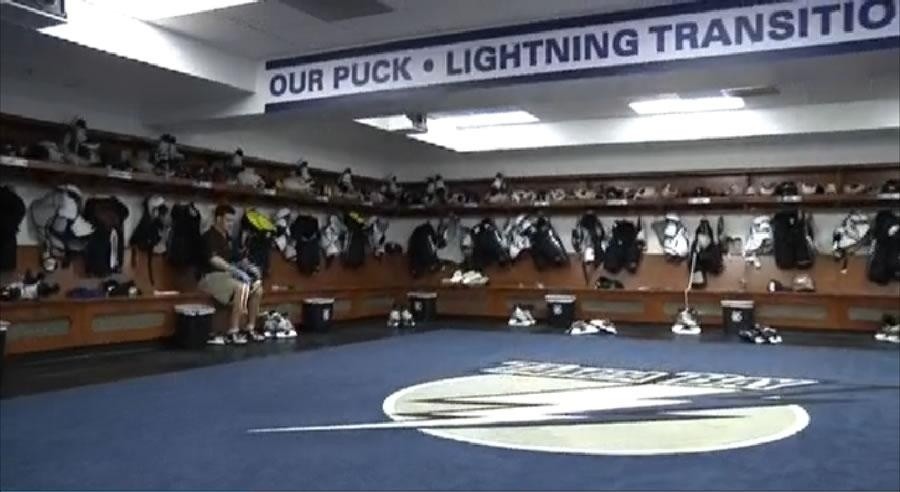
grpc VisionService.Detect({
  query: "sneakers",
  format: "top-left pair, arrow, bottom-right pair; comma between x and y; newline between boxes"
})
565,317 -> 618,336
873,321 -> 900,345
424,173 -> 445,198
508,304 -> 537,327
155,133 -> 184,160
295,161 -> 315,185
387,309 -> 417,327
228,149 -> 245,173
339,169 -> 352,191
208,310 -> 298,345
71,118 -> 101,150
490,172 -> 504,192
671,308 -> 702,335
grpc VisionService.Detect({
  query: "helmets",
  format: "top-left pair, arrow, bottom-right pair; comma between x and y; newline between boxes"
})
774,181 -> 797,196
881,179 -> 900,193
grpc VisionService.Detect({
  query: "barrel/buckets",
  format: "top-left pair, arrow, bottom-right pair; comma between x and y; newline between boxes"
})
174,302 -> 216,348
408,291 -> 439,320
299,297 -> 334,327
545,294 -> 575,328
721,300 -> 757,334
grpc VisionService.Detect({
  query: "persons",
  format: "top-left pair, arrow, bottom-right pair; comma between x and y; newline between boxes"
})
191,203 -> 266,345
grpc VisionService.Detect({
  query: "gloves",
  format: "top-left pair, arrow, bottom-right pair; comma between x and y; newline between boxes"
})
225,258 -> 262,283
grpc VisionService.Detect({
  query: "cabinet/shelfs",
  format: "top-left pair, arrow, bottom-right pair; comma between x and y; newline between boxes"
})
0,111 -> 900,357
